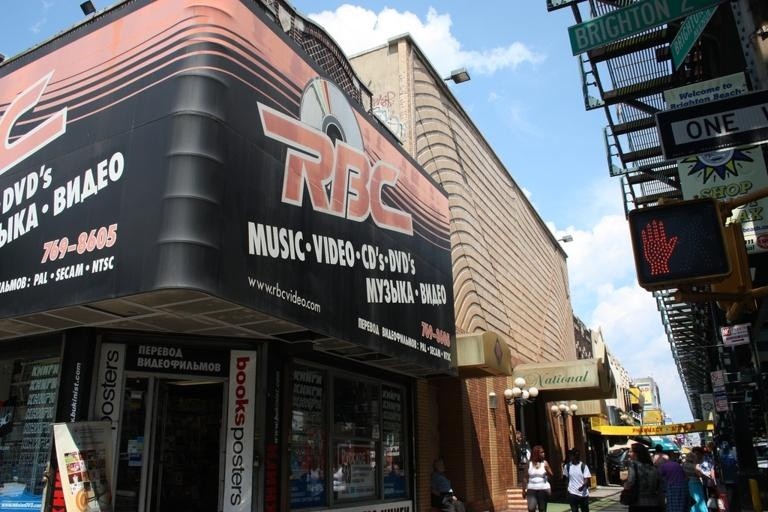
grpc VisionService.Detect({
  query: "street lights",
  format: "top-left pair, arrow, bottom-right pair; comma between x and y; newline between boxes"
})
505,375 -> 540,486
551,401 -> 578,472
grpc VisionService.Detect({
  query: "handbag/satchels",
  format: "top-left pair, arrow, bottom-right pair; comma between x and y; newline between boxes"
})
619,463 -> 640,506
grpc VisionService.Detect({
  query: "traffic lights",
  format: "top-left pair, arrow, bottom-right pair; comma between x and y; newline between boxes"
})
629,198 -> 734,292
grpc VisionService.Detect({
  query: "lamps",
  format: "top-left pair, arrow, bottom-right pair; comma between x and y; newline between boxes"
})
444,67 -> 470,85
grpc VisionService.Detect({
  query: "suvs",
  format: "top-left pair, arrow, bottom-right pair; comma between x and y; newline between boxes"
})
750,439 -> 767,476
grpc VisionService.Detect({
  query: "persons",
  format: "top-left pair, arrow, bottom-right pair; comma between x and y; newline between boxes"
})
619,443 -> 710,512
522,445 -> 554,512
431,459 -> 452,508
559,446 -> 592,512
